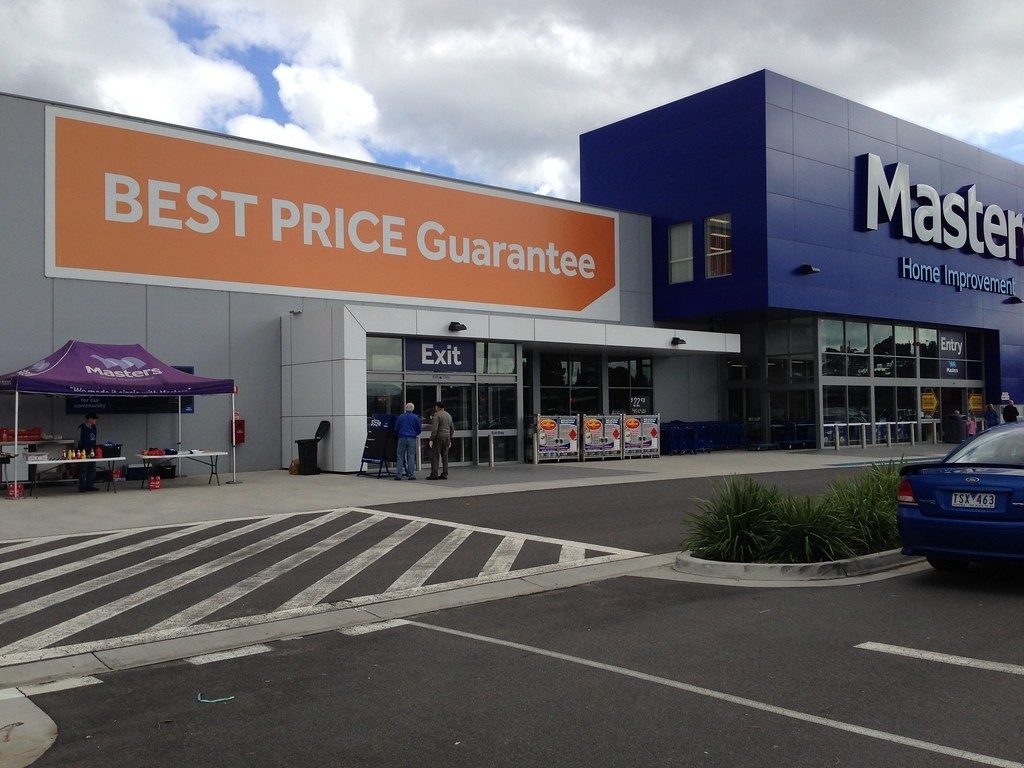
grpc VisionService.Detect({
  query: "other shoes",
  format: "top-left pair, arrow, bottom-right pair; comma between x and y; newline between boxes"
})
426,475 -> 438,480
88,487 -> 99,490
408,477 -> 417,480
438,475 -> 447,479
80,488 -> 86,492
394,477 -> 401,480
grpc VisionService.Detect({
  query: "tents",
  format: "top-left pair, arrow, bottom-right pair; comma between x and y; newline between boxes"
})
0,340 -> 235,498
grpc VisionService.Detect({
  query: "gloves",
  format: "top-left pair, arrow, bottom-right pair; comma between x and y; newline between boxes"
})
449,442 -> 451,448
429,441 -> 433,448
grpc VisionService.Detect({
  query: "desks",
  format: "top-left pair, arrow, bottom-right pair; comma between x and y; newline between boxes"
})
133,449 -> 228,491
22,456 -> 126,499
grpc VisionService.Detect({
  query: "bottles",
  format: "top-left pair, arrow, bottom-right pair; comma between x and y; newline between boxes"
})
90,447 -> 102,459
60,449 -> 86,460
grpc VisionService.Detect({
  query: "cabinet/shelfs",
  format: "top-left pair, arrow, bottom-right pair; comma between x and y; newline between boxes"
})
0,439 -> 74,483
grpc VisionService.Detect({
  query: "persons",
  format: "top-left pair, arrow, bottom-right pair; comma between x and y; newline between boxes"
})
74,412 -> 99,492
394,403 -> 422,480
967,416 -> 977,438
985,404 -> 998,428
1003,400 -> 1019,423
426,402 -> 455,480
953,410 -> 966,418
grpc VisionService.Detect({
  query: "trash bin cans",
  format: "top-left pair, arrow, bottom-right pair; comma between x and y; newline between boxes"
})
295,420 -> 331,475
944,415 -> 967,443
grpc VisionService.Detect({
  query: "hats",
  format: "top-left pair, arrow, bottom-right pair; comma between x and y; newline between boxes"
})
435,402 -> 446,408
406,403 -> 414,411
85,413 -> 99,419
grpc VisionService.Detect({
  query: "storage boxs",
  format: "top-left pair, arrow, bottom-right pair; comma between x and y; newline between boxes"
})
128,463 -> 152,481
38,472 -> 63,480
22,452 -> 49,461
96,443 -> 123,458
0,427 -> 43,442
157,464 -> 176,479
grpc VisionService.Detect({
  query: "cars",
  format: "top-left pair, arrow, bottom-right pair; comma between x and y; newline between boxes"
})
444,406 -> 533,450
897,420 -> 1024,572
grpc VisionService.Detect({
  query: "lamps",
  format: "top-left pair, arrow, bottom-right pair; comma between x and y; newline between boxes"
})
289,310 -> 302,315
799,264 -> 821,274
449,322 -> 468,332
1010,296 -> 1024,303
671,337 -> 686,345
